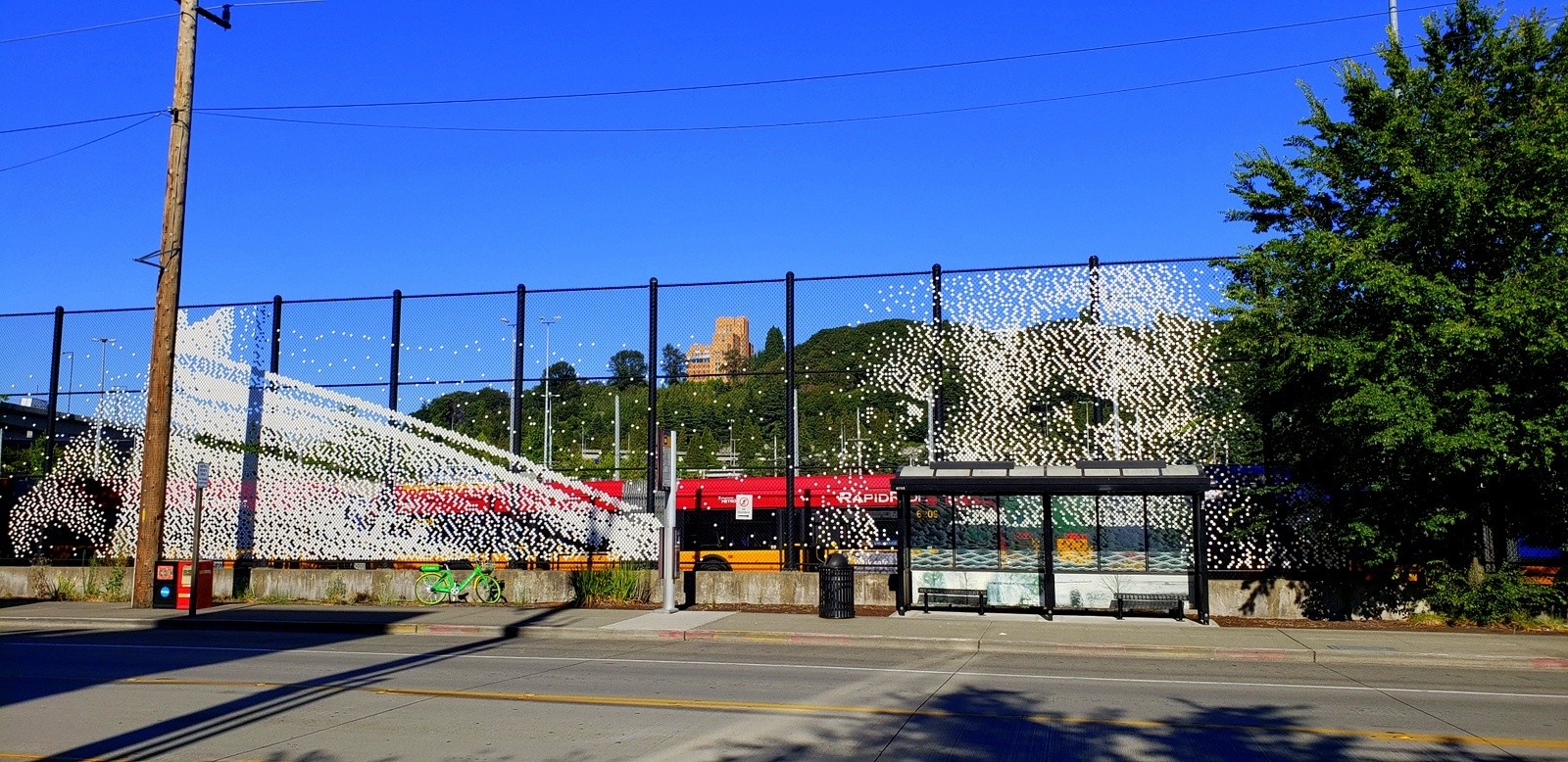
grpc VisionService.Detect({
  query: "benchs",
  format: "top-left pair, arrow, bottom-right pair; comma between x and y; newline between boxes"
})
1110,593 -> 1190,622
918,588 -> 987,615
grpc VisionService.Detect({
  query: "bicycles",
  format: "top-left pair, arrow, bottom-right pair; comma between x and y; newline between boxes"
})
414,552 -> 501,605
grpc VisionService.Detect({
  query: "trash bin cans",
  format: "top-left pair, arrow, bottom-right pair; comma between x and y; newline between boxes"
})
818,553 -> 856,619
153,560 -> 178,609
177,560 -> 213,610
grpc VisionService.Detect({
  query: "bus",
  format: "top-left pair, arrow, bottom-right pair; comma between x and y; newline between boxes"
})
678,508 -> 1089,571
